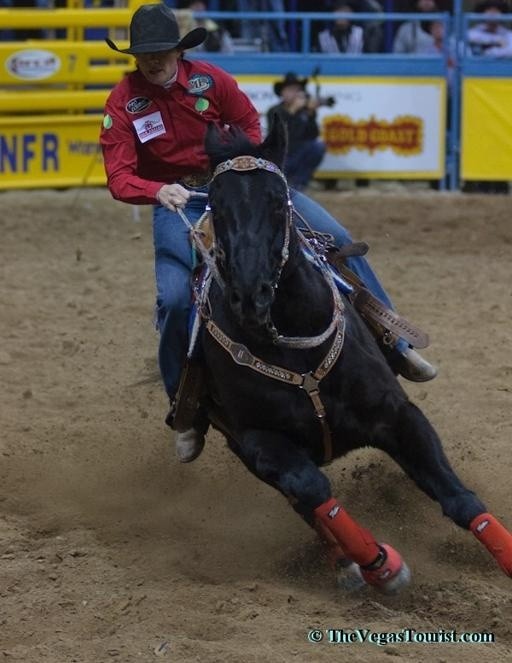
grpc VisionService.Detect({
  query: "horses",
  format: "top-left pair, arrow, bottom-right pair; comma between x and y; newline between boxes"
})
194,112 -> 512,596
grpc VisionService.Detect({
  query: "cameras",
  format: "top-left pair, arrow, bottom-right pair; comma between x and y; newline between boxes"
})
300,92 -> 334,108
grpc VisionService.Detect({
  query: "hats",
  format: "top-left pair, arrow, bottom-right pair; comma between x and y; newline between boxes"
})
104,3 -> 208,55
275,70 -> 308,96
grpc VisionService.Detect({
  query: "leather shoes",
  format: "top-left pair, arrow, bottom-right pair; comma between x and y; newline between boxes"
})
175,417 -> 212,462
389,346 -> 435,382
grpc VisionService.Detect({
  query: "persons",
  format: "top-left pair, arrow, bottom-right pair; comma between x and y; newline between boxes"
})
174,0 -> 511,58
98,2 -> 438,464
259,66 -> 336,193
